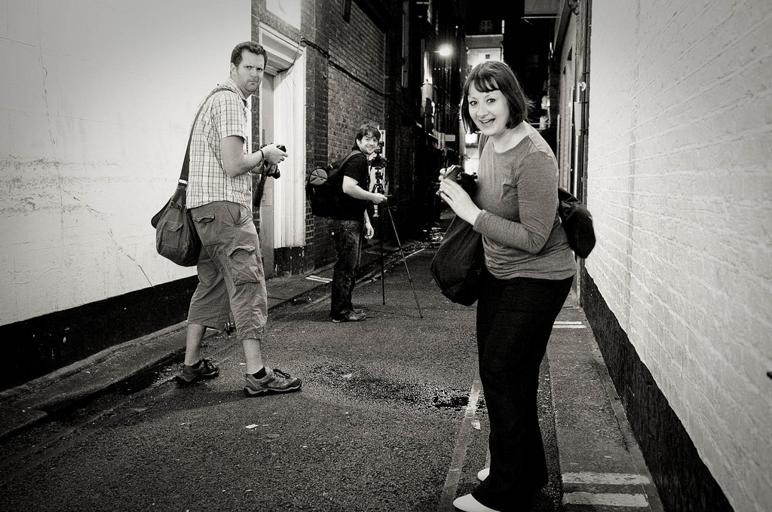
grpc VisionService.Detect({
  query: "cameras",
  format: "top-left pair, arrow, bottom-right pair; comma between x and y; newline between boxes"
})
371,141 -> 388,167
260,143 -> 286,179
435,166 -> 479,207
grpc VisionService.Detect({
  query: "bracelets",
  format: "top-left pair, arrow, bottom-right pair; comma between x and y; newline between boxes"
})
258,149 -> 264,162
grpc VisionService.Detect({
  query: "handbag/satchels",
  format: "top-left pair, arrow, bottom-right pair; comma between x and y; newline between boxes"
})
152,189 -> 201,267
557,188 -> 595,258
429,212 -> 486,306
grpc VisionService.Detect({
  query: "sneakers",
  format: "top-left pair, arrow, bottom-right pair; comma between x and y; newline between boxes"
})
452,493 -> 501,512
181,359 -> 221,385
243,368 -> 303,397
329,308 -> 366,324
477,467 -> 490,480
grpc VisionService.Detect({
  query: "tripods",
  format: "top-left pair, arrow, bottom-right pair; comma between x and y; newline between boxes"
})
371,172 -> 424,319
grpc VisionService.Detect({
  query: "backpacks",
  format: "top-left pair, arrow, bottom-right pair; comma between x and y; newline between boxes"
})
305,152 -> 364,220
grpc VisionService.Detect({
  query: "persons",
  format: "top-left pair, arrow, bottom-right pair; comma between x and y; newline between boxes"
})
329,124 -> 388,322
439,60 -> 577,512
175,41 -> 302,397
